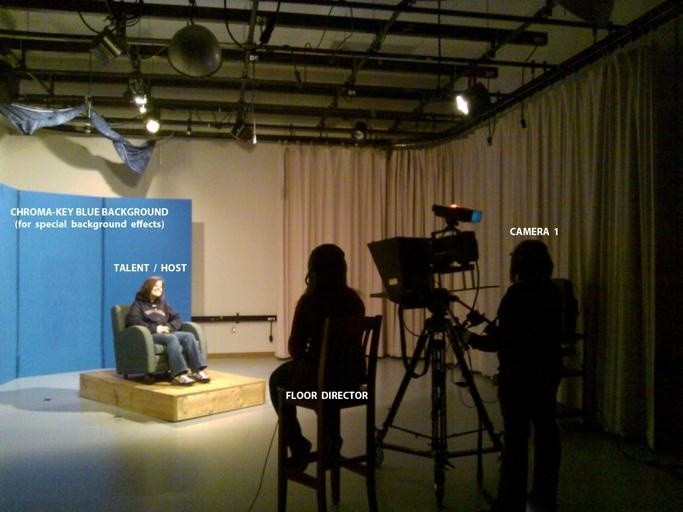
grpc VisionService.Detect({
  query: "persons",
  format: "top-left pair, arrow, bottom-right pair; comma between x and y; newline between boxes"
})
267,243 -> 366,475
463,236 -> 579,512
125,275 -> 211,386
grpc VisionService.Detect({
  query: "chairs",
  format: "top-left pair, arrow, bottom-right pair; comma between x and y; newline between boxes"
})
275,316 -> 384,511
112,304 -> 207,384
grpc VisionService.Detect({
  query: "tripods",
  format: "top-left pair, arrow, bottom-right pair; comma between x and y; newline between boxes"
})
376,312 -> 503,512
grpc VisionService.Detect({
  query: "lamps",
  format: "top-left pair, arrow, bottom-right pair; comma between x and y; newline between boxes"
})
165,1 -> 224,79
351,122 -> 368,142
129,71 -> 161,136
455,63 -> 490,116
96,21 -> 125,61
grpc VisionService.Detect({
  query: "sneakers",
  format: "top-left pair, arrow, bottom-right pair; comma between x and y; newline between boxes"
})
190,370 -> 210,384
172,375 -> 194,386
285,442 -> 311,480
